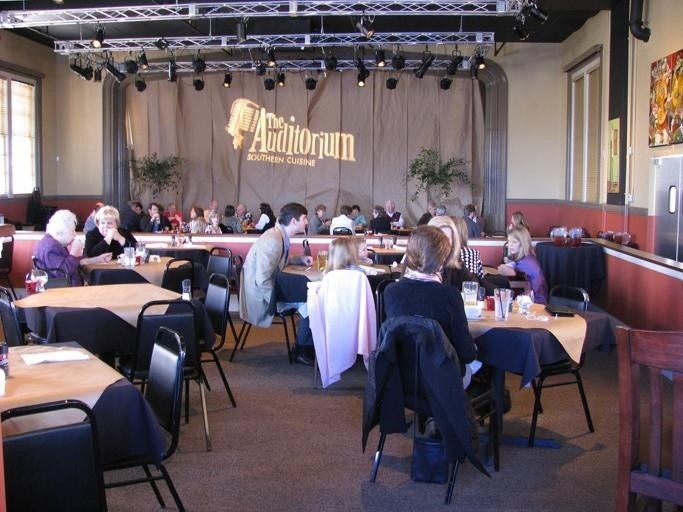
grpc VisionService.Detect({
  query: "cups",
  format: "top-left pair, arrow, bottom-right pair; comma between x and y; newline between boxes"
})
463,282 -> 534,321
397,226 -> 400,230
317,251 -> 328,272
550,226 -> 583,248
368,231 -> 372,237
124,247 -> 135,269
136,243 -> 146,260
613,232 -> 624,244
392,226 -> 396,230
605,231 -> 615,241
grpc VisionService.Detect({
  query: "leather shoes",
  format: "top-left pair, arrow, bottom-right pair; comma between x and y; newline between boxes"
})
422,417 -> 440,437
297,356 -> 312,365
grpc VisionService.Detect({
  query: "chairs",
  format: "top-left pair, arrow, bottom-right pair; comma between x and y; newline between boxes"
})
613,326 -> 683,511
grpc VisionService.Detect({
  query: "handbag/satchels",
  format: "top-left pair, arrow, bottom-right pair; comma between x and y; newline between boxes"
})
410,438 -> 448,483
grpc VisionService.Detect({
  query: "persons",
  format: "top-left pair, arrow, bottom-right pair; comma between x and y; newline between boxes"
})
33,209 -> 112,286
237,202 -> 318,366
308,199 -> 530,238
83,200 -> 275,237
323,215 -> 548,439
83,205 -> 138,261
